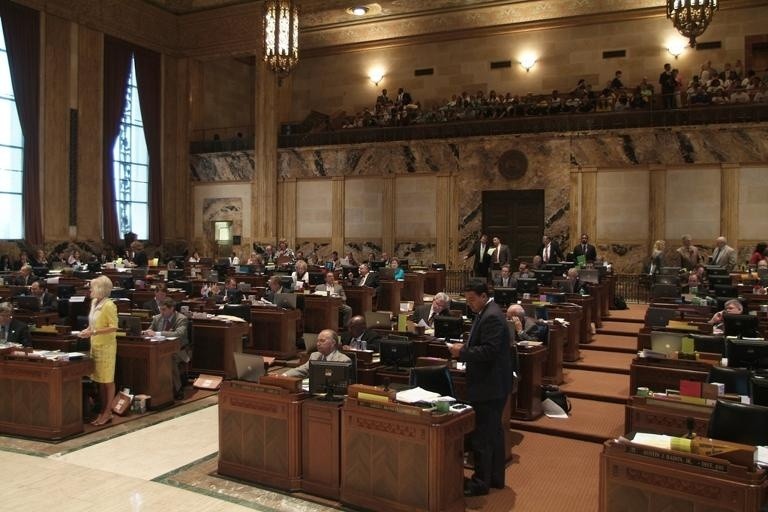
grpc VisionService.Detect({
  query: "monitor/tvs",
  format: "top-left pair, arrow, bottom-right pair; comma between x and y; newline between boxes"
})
375,340 -> 416,375
433,315 -> 466,344
506,319 -> 516,344
517,277 -> 539,299
645,262 -> 767,450
558,261 -> 575,273
494,287 -> 519,313
365,312 -> 392,330
308,359 -> 354,405
1,258 -> 296,336
533,269 -> 553,288
545,264 -> 566,280
449,299 -> 468,316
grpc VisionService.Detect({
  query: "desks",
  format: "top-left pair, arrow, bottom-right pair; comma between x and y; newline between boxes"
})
219,258 -> 608,510
599,270 -> 767,512
0,261 -> 445,444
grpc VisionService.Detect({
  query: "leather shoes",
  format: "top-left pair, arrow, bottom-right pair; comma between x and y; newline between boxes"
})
178,389 -> 184,399
464,476 -> 504,496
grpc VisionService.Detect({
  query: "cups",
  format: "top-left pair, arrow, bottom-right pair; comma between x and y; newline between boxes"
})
751,286 -> 768,312
360,340 -> 367,351
431,399 -> 450,413
690,287 -> 701,305
0,278 -> 4,286
637,387 -> 649,397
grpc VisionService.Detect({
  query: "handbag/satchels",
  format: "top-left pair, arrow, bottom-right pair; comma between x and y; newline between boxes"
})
543,391 -> 571,413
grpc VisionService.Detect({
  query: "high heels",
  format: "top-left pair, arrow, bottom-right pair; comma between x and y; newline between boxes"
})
91,415 -> 113,425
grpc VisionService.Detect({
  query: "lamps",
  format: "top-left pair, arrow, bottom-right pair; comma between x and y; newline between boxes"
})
666,40 -> 684,59
661,1 -> 721,50
518,56 -> 536,76
260,1 -> 303,89
367,69 -> 386,86
349,6 -> 369,17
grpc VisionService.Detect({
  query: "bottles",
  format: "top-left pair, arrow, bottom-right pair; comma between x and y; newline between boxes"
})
579,287 -> 584,295
549,298 -> 552,304
688,433 -> 701,455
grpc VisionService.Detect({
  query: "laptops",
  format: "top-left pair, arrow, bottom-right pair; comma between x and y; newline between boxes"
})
231,351 -> 285,382
